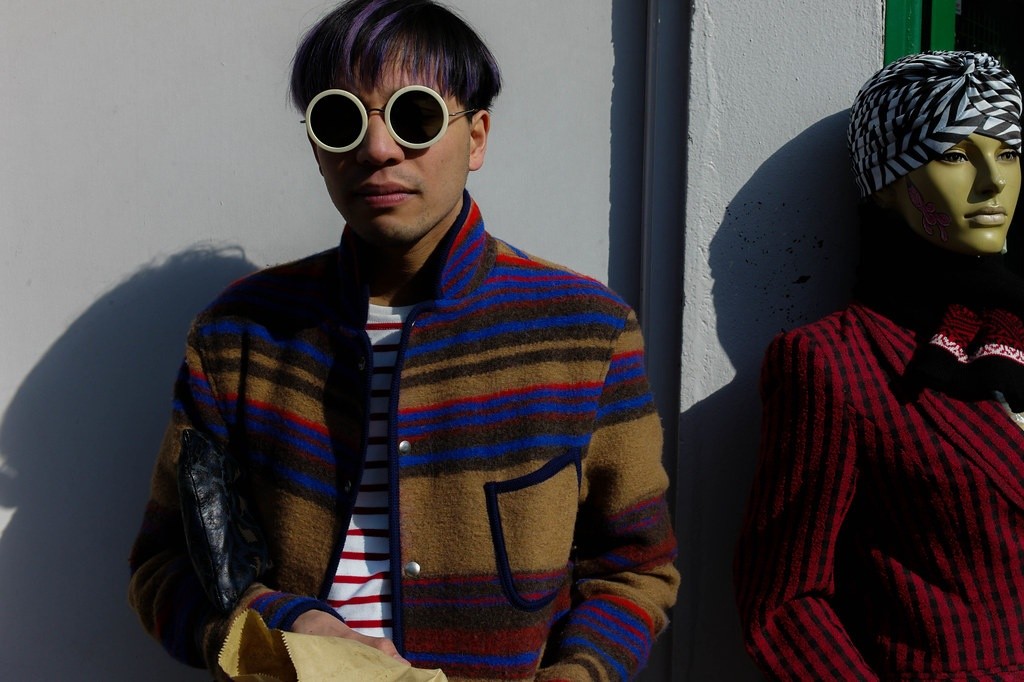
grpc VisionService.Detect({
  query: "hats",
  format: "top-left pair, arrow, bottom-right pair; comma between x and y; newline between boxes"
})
847,48 -> 1022,199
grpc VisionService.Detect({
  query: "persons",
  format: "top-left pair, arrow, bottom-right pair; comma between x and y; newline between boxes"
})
707,49 -> 1024,682
126,0 -> 686,681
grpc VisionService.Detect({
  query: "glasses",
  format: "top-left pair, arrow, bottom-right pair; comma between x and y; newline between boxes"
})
300,84 -> 475,153
